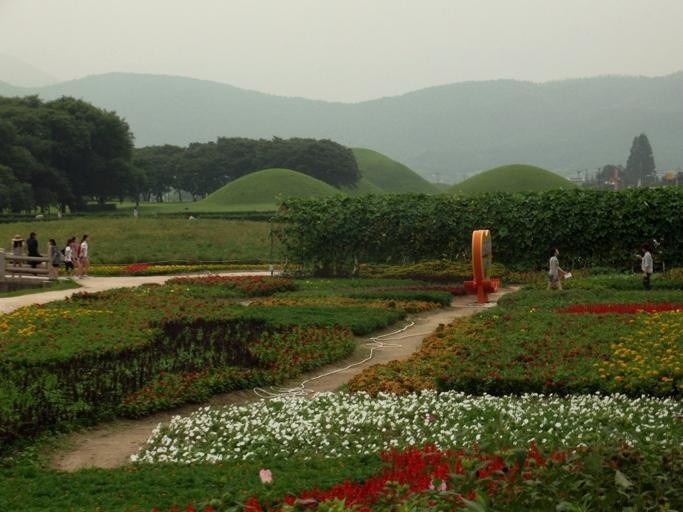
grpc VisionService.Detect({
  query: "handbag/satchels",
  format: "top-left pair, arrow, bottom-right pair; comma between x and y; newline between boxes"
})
547,272 -> 554,280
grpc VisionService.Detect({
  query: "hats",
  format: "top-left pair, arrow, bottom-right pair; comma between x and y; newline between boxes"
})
11,234 -> 25,242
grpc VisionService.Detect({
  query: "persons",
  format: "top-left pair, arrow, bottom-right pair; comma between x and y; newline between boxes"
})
547,248 -> 567,291
11,232 -> 90,280
635,242 -> 653,291
